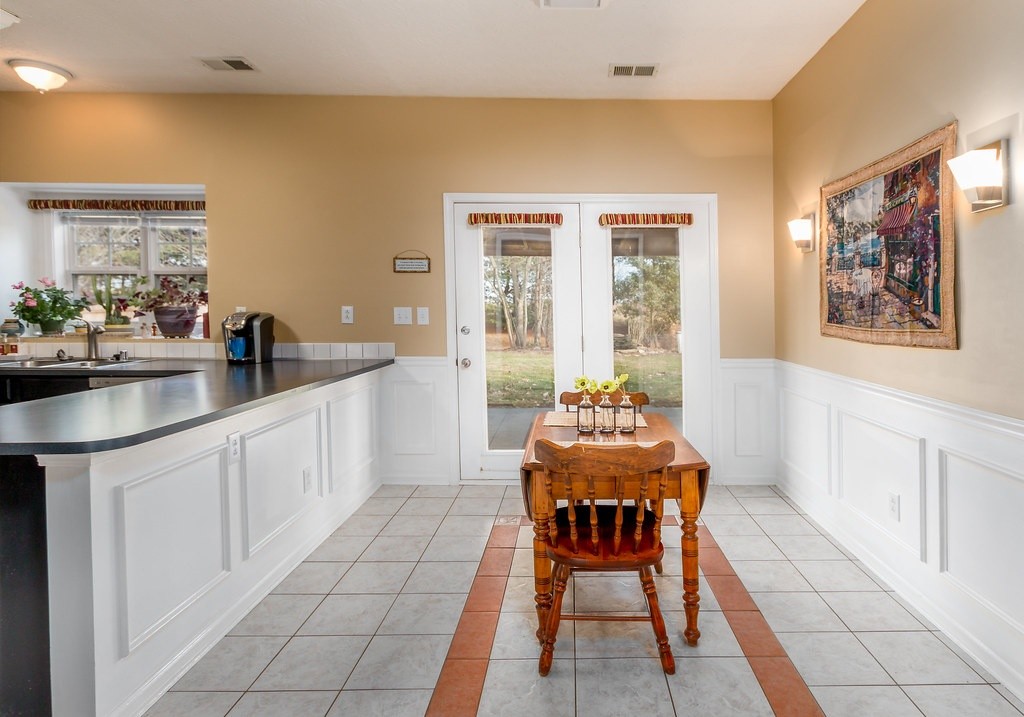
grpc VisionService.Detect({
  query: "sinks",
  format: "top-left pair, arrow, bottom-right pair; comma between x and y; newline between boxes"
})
0,359 -> 75,367
49,360 -> 132,368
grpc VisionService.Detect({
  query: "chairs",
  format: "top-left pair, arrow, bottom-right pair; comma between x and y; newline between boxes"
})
559,390 -> 650,415
535,439 -> 676,675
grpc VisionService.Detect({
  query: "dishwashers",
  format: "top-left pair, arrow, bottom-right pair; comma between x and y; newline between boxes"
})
88,376 -> 173,390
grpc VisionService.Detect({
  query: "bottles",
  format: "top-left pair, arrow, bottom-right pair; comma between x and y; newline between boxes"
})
151,322 -> 157,336
142,322 -> 149,338
0,332 -> 21,355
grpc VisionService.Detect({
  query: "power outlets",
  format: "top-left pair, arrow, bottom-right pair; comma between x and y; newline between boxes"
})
227,431 -> 241,464
394,307 -> 413,325
303,466 -> 313,493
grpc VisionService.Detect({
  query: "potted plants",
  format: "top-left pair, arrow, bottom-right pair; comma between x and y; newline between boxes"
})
93,274 -> 134,340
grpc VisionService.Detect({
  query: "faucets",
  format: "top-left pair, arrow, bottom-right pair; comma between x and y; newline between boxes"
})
74,315 -> 106,360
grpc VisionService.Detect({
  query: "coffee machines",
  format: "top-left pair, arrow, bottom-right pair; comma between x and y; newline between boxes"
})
221,311 -> 275,366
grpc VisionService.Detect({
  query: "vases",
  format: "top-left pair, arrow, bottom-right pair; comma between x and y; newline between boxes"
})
578,395 -> 594,433
620,396 -> 635,433
154,307 -> 198,338
39,320 -> 64,336
599,395 -> 615,432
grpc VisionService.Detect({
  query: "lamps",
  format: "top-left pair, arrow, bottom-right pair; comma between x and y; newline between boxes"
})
9,60 -> 72,94
947,149 -> 1009,211
787,213 -> 814,254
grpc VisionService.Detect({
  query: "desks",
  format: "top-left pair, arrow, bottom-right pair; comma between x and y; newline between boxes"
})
520,411 -> 711,648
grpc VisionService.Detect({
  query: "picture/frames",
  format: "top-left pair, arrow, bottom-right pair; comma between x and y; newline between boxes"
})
820,119 -> 959,349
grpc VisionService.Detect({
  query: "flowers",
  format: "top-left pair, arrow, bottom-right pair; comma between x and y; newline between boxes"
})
575,375 -> 589,395
9,279 -> 92,322
613,374 -> 629,397
117,276 -> 209,317
589,379 -> 617,396
